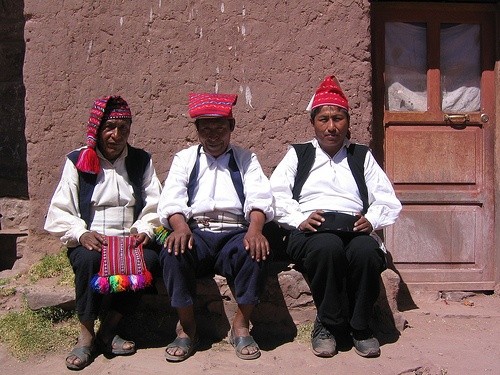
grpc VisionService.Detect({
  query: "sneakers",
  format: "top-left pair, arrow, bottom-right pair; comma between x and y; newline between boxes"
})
309,313 -> 336,359
350,324 -> 380,357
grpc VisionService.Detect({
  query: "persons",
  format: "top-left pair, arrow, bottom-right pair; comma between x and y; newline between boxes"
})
269,75 -> 402,357
43,96 -> 162,370
157,94 -> 275,359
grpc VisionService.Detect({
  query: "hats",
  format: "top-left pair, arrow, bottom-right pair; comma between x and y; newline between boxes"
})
77,96 -> 133,174
305,75 -> 348,111
188,91 -> 238,121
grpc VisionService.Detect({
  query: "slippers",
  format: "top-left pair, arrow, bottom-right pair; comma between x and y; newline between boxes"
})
66,338 -> 96,370
165,334 -> 200,361
229,328 -> 260,359
96,330 -> 136,355
